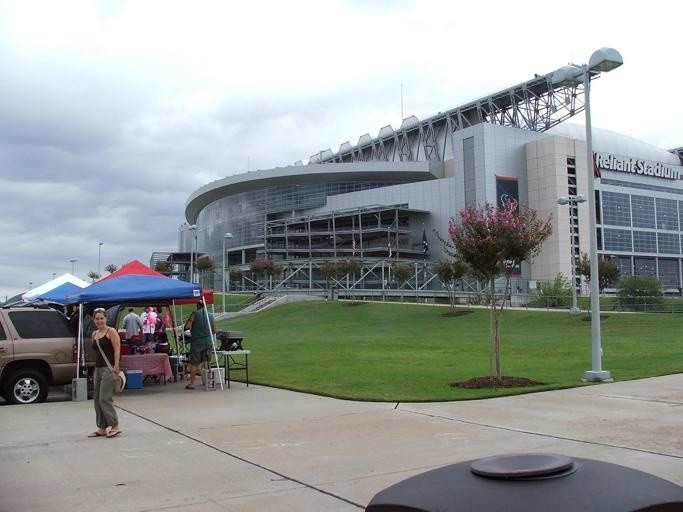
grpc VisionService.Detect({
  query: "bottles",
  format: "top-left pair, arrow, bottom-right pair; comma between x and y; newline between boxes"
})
132,344 -> 145,355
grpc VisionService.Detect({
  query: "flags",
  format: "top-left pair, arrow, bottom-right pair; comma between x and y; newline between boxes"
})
352,238 -> 356,256
422,226 -> 428,254
388,231 -> 393,258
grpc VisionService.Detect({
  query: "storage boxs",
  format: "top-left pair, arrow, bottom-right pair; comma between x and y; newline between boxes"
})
125,368 -> 143,389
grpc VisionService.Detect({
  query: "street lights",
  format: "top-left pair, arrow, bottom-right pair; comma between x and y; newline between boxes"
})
188,226 -> 194,284
69,259 -> 77,274
192,225 -> 197,284
98,241 -> 104,278
550,48 -> 624,382
557,193 -> 587,313
222,232 -> 234,315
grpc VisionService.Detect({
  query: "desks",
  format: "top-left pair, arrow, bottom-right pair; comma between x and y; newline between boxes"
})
182,350 -> 249,390
120,354 -> 171,385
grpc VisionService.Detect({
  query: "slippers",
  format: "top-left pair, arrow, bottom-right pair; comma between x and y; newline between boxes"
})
185,384 -> 195,389
88,432 -> 107,437
107,431 -> 121,438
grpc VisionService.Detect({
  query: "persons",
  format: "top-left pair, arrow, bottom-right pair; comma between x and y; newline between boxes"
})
184,298 -> 217,389
88,307 -> 122,439
122,306 -> 170,356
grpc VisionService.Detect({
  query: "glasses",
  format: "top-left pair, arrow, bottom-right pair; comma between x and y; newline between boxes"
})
93,308 -> 106,318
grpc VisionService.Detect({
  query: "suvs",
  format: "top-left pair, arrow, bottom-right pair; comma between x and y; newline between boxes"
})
1,300 -> 79,404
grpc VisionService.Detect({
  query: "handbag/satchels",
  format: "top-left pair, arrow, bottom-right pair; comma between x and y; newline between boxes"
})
114,371 -> 126,393
